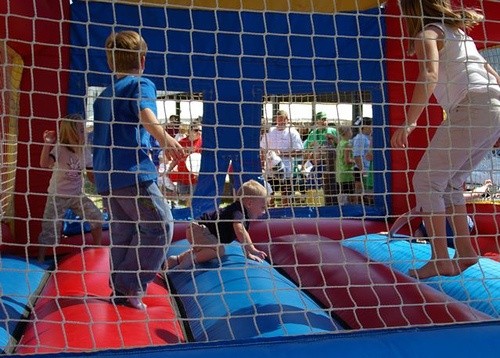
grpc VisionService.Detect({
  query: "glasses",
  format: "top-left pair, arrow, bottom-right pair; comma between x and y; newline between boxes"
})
192,129 -> 202,132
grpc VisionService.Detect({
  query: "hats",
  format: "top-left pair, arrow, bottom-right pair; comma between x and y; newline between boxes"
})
316,112 -> 328,121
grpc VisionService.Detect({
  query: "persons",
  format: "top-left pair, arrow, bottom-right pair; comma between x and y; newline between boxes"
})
379,179 -> 500,248
390,0 -> 500,279
260,110 -> 374,206
85,132 -> 96,184
156,115 -> 202,205
37,115 -> 104,261
93,31 -> 184,310
162,181 -> 268,271
